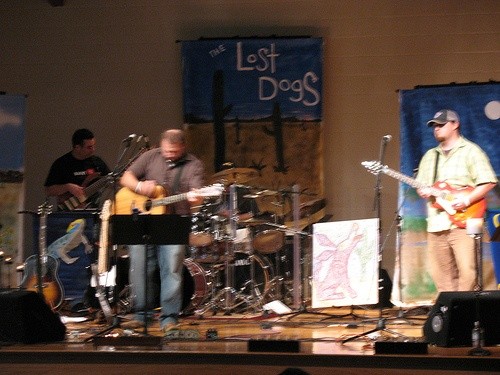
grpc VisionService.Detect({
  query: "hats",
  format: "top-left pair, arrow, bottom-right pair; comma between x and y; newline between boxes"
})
427,110 -> 459,128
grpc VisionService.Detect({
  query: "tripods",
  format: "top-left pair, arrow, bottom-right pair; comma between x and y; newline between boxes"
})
83,140 -> 149,342
195,143 -> 416,344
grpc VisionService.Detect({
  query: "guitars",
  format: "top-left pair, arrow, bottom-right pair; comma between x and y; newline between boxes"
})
360,159 -> 488,229
45,167 -> 121,212
109,181 -> 226,214
17,200 -> 64,310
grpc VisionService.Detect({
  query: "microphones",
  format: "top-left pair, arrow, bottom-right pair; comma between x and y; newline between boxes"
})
383,135 -> 392,144
121,133 -> 136,142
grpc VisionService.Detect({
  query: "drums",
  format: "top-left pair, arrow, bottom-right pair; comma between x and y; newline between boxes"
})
146,215 -> 286,317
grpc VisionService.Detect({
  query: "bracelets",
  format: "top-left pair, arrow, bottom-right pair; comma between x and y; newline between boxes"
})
463,196 -> 471,208
134,181 -> 142,193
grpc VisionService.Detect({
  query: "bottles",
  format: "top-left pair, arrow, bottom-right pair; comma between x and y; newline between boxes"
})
472,321 -> 484,349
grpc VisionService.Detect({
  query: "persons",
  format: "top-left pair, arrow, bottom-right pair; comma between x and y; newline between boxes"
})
414,109 -> 499,303
43,129 -> 112,209
118,129 -> 204,332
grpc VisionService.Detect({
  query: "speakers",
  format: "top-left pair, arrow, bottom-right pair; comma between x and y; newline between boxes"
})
423,290 -> 500,348
0,287 -> 66,344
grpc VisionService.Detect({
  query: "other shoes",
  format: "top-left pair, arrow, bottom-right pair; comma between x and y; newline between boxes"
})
72,303 -> 91,312
162,323 -> 178,332
120,319 -> 144,328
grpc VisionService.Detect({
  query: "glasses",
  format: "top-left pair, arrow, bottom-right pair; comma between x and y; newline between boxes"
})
433,122 -> 447,128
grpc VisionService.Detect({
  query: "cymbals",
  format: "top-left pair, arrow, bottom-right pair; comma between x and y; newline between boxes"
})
208,167 -> 260,188
283,198 -> 327,222
